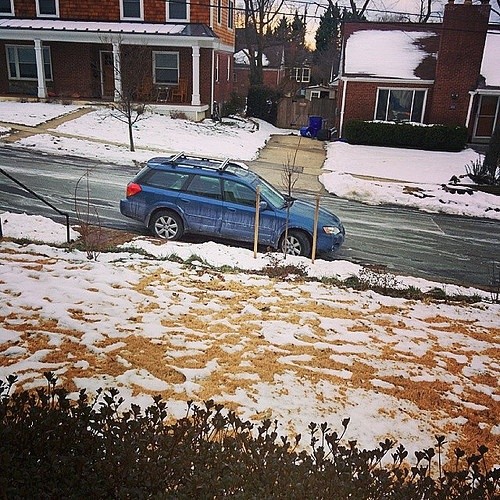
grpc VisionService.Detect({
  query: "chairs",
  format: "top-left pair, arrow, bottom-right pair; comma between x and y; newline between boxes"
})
173,79 -> 188,104
135,80 -> 152,102
228,181 -> 242,204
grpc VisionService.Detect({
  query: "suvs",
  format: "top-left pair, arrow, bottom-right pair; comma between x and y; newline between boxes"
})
120,151 -> 345,260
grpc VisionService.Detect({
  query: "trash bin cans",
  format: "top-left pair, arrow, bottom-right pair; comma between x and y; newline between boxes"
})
309,116 -> 323,138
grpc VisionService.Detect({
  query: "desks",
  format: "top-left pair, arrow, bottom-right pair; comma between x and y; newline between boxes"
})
156,86 -> 173,104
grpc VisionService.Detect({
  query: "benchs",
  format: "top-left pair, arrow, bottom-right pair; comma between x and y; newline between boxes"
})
173,178 -> 202,196
